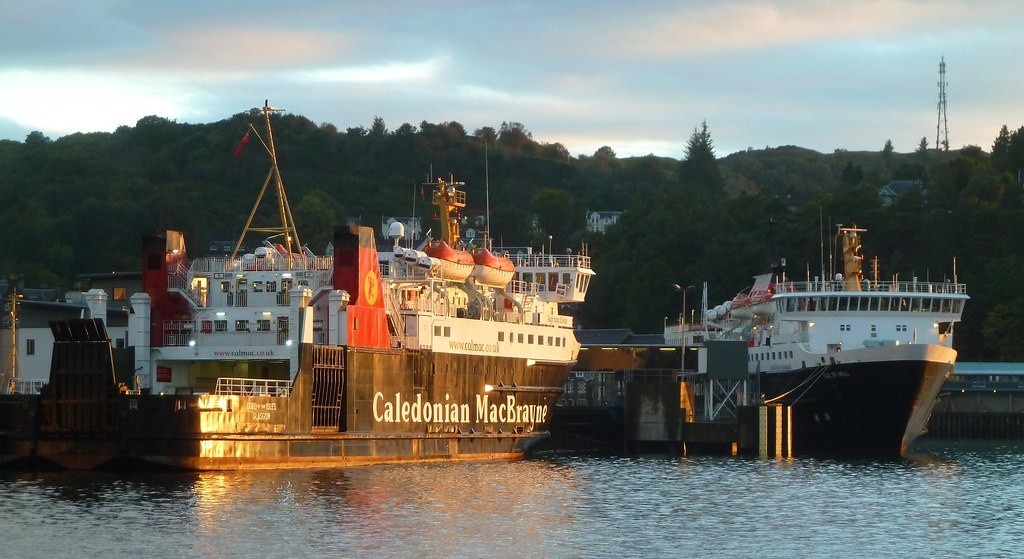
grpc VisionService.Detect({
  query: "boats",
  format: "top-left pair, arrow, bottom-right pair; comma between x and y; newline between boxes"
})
146,99 -> 597,467
664,228 -> 971,458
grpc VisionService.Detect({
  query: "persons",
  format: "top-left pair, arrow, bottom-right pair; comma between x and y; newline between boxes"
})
118,381 -> 128,394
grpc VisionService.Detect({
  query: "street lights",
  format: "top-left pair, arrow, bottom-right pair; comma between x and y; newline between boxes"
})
673,282 -> 696,440
